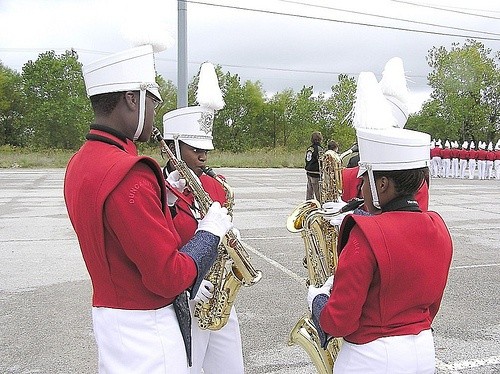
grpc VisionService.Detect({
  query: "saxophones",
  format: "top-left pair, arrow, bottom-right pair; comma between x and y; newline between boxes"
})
288,197 -> 365,374
193,165 -> 246,331
286,144 -> 359,289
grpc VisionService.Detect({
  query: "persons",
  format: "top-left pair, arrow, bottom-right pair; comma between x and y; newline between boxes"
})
429,139 -> 500,179
306,128 -> 454,374
324,139 -> 341,164
321,97 -> 431,230
153,105 -> 245,373
63,44 -> 233,374
305,130 -> 324,203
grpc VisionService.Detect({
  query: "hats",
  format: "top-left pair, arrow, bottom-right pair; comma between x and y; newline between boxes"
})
430,137 -> 500,150
163,60 -> 226,151
357,72 -> 431,176
82,43 -> 165,103
378,56 -> 410,131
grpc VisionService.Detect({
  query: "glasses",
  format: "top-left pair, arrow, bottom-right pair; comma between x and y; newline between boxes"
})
132,92 -> 160,108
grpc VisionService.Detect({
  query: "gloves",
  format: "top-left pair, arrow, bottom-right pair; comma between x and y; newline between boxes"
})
323,213 -> 347,228
307,275 -> 335,313
323,196 -> 354,214
165,169 -> 202,206
195,201 -> 234,246
187,279 -> 215,305
229,227 -> 241,244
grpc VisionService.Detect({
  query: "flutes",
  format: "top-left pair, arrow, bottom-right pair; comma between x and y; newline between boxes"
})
152,127 -> 263,287
